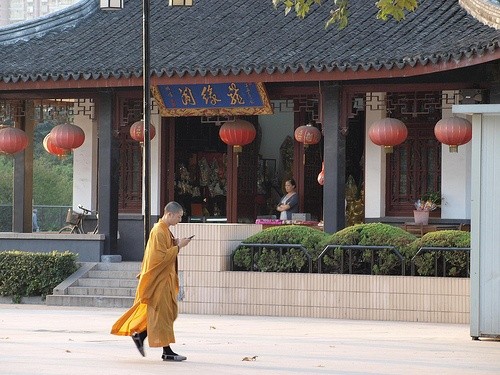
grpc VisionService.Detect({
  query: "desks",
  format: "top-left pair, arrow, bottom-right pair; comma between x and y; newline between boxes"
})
407,224 -> 461,234
255,218 -> 319,229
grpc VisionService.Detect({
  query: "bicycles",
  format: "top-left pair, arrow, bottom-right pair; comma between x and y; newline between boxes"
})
57,205 -> 121,254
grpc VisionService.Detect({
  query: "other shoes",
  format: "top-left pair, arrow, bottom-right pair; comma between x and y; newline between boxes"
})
132,332 -> 144,357
162,354 -> 186,361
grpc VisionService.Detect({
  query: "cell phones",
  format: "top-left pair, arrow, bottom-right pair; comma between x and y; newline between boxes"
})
189,235 -> 194,239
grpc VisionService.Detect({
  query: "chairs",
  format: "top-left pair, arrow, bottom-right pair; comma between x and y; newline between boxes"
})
405,222 -> 423,238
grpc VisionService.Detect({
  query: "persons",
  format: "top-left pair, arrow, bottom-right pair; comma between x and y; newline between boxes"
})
276,178 -> 299,220
33,209 -> 39,231
317,162 -> 347,227
110,201 -> 192,361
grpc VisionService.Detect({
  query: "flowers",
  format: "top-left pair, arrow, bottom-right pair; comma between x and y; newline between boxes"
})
413,191 -> 445,211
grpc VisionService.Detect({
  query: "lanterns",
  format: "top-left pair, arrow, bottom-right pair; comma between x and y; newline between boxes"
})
219,119 -> 256,152
434,116 -> 472,152
130,119 -> 156,148
295,125 -> 321,148
50,122 -> 85,155
43,133 -> 71,156
369,118 -> 407,153
0,126 -> 29,155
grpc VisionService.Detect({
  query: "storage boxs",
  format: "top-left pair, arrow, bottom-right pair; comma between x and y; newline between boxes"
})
292,213 -> 311,221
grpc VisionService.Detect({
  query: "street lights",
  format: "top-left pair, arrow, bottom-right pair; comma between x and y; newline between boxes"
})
99,0 -> 194,252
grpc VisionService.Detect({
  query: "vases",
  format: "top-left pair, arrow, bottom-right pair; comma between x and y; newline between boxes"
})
414,210 -> 429,225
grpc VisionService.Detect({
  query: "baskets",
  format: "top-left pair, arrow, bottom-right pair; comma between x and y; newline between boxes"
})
66,208 -> 82,225
